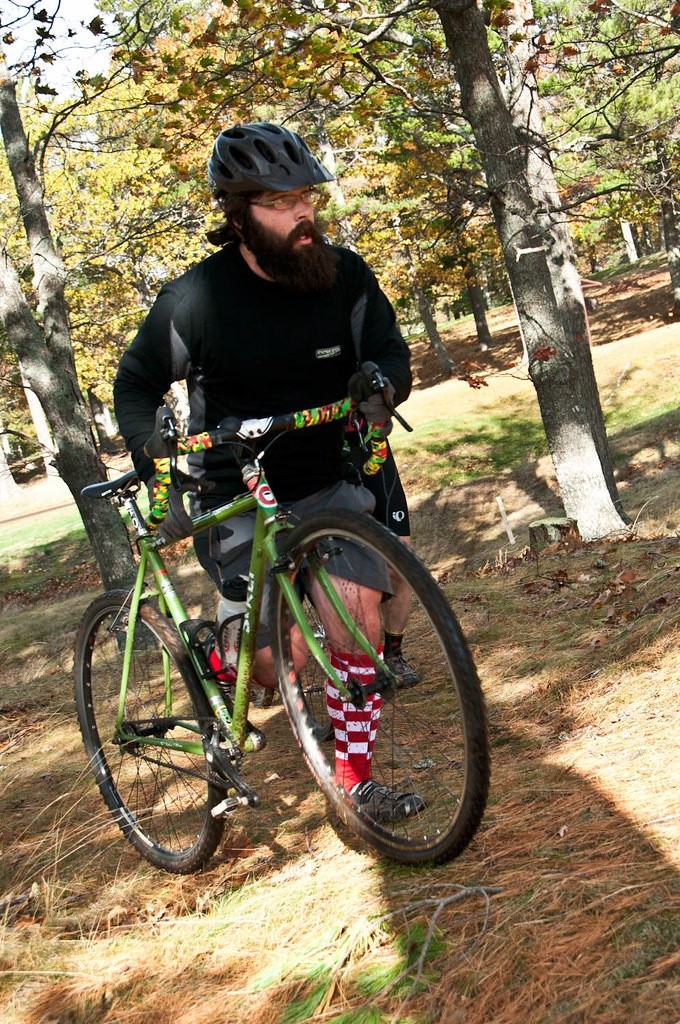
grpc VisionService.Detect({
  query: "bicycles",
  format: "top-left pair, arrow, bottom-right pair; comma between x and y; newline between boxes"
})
69,364 -> 493,877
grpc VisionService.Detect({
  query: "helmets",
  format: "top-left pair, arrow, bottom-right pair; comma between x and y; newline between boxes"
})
209,123 -> 337,193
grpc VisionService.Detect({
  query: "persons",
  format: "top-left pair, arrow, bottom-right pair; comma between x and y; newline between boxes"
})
108,124 -> 429,826
339,439 -> 418,696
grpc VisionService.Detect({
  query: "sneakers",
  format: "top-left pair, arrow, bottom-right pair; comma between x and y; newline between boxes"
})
388,657 -> 418,690
350,783 -> 423,818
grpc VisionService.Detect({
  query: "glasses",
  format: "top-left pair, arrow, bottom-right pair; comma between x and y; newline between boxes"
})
246,190 -> 320,209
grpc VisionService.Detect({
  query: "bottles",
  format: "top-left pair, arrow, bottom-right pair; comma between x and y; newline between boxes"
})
210,573 -> 248,684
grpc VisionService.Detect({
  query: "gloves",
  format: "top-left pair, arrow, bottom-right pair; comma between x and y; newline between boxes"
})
149,467 -> 203,539
362,384 -> 397,438
